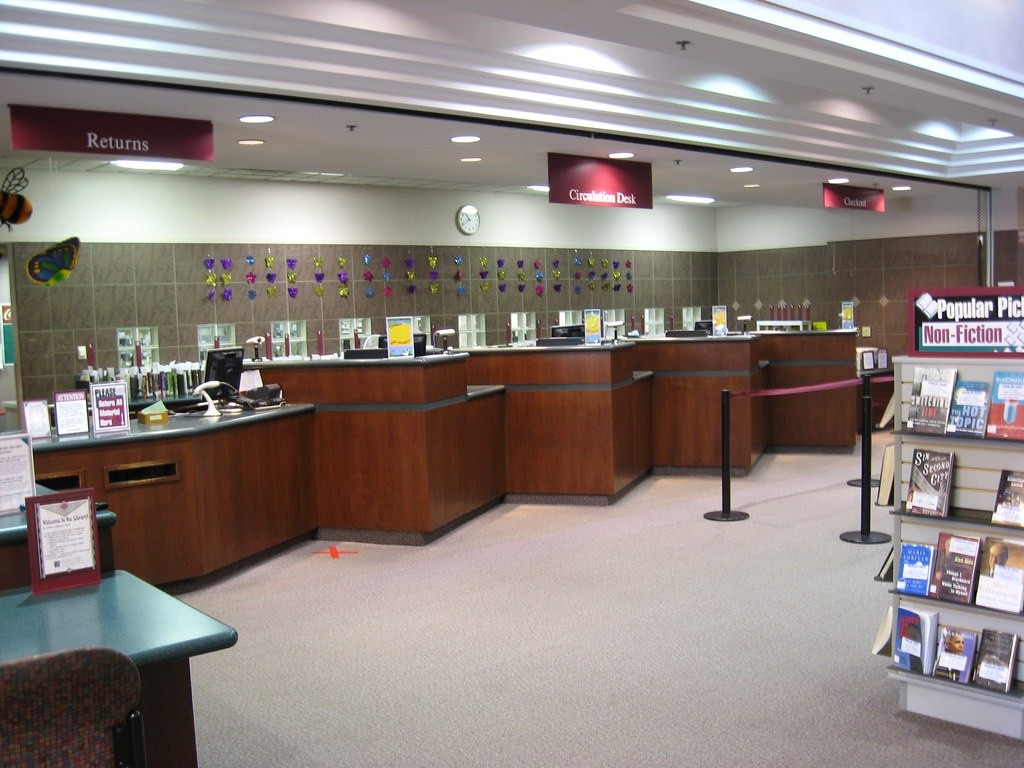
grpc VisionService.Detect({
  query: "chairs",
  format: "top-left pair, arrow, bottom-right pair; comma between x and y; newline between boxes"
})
0,647 -> 147,768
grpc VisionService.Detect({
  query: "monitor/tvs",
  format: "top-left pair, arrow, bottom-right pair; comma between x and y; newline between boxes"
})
551,325 -> 585,338
379,334 -> 427,357
201,347 -> 244,401
694,320 -> 713,336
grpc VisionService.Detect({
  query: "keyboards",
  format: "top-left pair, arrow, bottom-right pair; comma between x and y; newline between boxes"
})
174,400 -> 218,414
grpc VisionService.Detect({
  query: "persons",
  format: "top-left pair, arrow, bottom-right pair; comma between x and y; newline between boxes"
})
935,630 -> 964,682
981,543 -> 1007,575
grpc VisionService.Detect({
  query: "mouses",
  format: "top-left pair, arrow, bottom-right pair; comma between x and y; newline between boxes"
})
167,410 -> 175,415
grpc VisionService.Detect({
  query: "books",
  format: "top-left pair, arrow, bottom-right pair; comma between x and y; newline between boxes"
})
872,606 -> 1020,693
79,360 -> 205,399
990,470 -> 1024,529
876,443 -> 895,505
905,449 -> 955,517
880,368 -> 1024,440
878,537 -> 1024,615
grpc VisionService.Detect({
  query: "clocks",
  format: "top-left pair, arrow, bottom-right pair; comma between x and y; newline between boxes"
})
456,204 -> 480,235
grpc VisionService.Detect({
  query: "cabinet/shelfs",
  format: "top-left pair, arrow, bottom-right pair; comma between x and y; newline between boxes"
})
887,354 -> 1023,740
